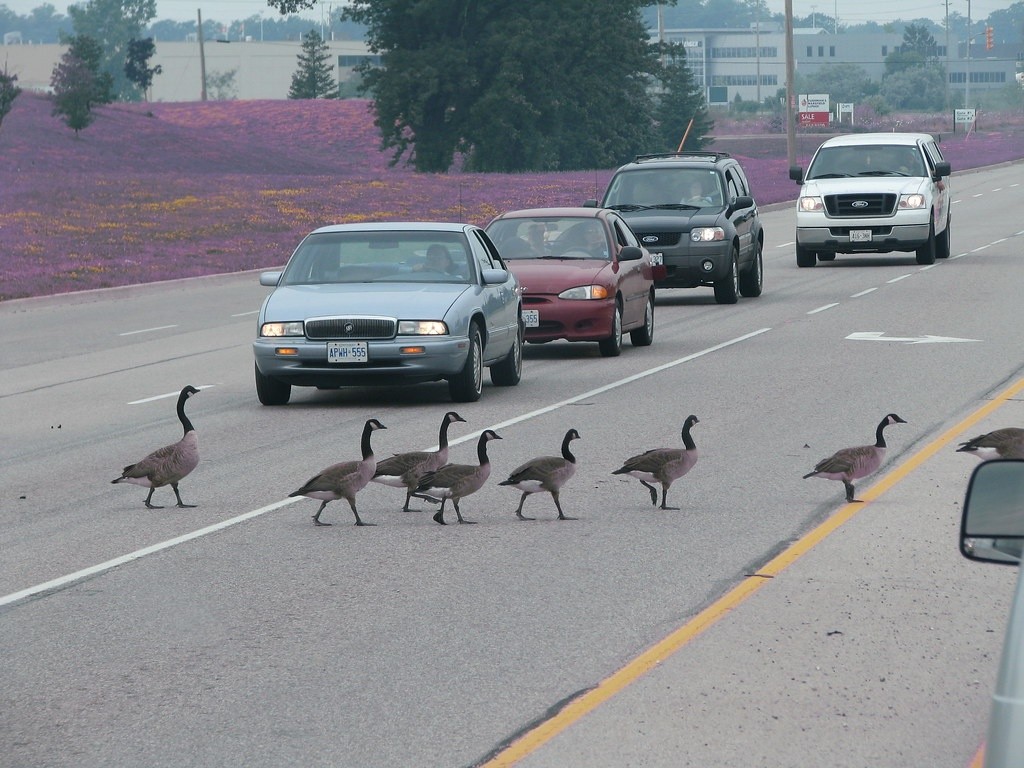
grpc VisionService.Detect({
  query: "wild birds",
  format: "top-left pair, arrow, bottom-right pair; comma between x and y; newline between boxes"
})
803,414 -> 907,503
956,427 -> 1024,459
288,412 -> 582,525
111,385 -> 201,510
611,415 -> 701,511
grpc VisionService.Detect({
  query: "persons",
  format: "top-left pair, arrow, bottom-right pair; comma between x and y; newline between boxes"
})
580,220 -> 622,259
526,223 -> 552,256
679,178 -> 712,206
898,150 -> 923,177
397,243 -> 469,282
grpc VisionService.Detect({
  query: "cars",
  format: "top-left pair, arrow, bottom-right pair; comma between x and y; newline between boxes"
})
483,207 -> 657,357
252,222 -> 525,402
959,455 -> 1024,767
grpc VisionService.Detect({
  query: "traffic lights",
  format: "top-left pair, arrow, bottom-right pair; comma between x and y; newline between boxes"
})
986,27 -> 994,50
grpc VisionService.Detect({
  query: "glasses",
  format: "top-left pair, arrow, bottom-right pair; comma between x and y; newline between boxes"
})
584,227 -> 602,234
427,254 -> 445,261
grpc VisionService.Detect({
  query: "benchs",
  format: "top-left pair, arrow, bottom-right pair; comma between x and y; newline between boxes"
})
363,262 -> 414,277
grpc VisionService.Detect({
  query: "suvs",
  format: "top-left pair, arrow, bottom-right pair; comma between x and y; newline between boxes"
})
583,151 -> 764,304
789,132 -> 951,264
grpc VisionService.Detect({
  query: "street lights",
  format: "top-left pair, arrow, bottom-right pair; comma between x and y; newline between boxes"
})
752,26 -> 760,104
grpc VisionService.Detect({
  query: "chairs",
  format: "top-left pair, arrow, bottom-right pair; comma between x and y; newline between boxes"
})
339,262 -> 377,282
633,183 -> 654,203
500,237 -> 529,256
